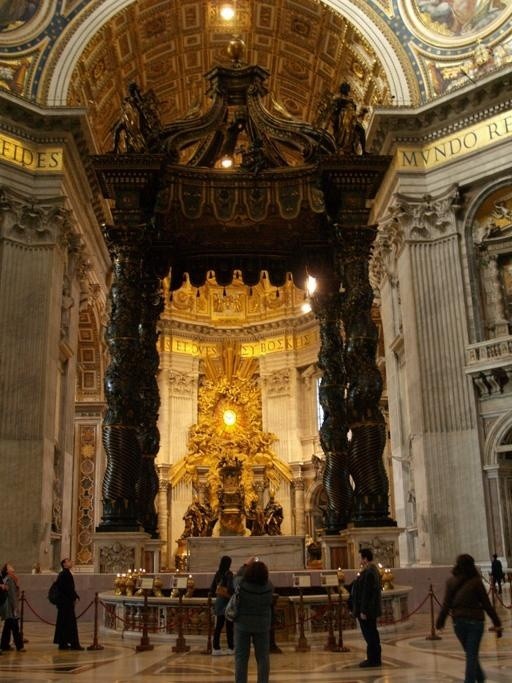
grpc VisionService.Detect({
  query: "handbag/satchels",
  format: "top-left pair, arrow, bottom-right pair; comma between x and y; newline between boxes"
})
222,577 -> 248,622
212,569 -> 238,601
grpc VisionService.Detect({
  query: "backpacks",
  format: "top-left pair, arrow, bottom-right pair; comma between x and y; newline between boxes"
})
47,569 -> 69,605
346,566 -> 382,619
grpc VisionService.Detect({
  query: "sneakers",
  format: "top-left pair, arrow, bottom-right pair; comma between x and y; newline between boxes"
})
17,646 -> 28,652
225,645 -> 236,656
68,643 -> 86,652
1,645 -> 15,651
57,642 -> 72,650
210,647 -> 226,656
357,657 -> 383,668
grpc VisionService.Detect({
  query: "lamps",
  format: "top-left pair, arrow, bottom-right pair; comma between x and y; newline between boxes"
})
112,567 -> 146,595
356,561 -> 395,592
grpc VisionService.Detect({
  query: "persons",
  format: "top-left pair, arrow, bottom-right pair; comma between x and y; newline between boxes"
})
435,553 -> 502,683
182,492 -> 284,536
0,563 -> 26,652
350,549 -> 381,667
210,555 -> 235,656
491,554 -> 505,594
52,558 -> 85,650
231,555 -> 270,683
